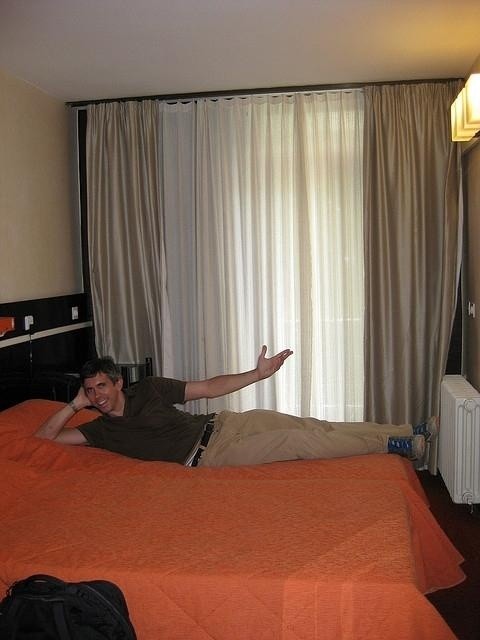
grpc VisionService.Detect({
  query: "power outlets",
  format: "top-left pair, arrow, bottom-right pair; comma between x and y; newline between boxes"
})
25,316 -> 33,331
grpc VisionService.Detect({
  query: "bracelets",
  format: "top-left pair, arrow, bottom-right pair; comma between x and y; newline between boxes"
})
68,401 -> 79,414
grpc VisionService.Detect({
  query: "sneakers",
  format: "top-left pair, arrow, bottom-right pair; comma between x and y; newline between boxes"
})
414,416 -> 438,440
387,435 -> 425,459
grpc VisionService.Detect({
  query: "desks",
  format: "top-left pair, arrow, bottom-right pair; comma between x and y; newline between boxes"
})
117,357 -> 153,390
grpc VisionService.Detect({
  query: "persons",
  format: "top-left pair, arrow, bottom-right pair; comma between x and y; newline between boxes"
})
31,344 -> 442,470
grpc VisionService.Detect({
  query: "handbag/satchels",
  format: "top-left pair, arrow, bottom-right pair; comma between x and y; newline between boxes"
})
0,574 -> 136,640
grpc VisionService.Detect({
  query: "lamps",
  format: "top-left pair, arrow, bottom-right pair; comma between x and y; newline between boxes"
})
451,73 -> 480,142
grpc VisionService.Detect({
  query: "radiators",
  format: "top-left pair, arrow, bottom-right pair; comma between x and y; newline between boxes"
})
438,375 -> 480,514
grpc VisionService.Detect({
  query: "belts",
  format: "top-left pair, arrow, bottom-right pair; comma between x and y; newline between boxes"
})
191,413 -> 215,466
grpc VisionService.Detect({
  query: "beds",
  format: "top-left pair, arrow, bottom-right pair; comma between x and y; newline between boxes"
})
0,293 -> 467,640
